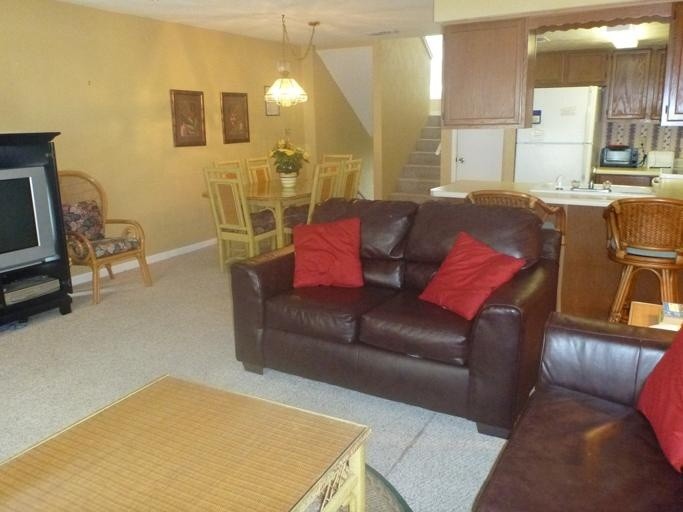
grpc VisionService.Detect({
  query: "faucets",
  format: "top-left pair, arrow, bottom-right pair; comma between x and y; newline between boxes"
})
554,174 -> 564,191
588,178 -> 595,190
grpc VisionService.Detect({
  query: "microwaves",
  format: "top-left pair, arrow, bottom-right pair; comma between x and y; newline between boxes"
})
600,148 -> 638,167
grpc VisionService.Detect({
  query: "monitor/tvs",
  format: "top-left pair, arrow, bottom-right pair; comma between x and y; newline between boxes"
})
0,166 -> 63,273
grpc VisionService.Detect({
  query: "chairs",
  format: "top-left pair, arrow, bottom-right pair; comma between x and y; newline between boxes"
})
462,190 -> 568,313
601,197 -> 683,326
204,154 -> 361,273
57,170 -> 153,303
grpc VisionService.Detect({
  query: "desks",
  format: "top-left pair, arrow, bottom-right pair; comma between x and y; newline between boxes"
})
627,299 -> 683,332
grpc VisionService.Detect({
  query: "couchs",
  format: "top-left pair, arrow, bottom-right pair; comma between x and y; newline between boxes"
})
472,313 -> 683,512
229,197 -> 560,438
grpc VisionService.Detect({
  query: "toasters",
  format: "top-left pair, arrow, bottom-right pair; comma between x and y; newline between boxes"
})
647,151 -> 674,168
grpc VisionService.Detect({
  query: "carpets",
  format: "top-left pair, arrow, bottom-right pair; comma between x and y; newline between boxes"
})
362,465 -> 413,512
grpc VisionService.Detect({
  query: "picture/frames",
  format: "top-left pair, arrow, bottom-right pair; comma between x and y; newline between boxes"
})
170,89 -> 207,148
221,93 -> 250,145
263,85 -> 280,116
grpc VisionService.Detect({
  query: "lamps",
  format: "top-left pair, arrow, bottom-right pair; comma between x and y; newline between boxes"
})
264,15 -> 309,107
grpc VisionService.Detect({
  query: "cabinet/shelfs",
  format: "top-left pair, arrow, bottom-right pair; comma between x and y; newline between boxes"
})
596,175 -> 650,186
439,4 -> 683,127
1,131 -> 73,324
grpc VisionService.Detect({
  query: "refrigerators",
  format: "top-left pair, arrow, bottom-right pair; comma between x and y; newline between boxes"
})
514,85 -> 604,184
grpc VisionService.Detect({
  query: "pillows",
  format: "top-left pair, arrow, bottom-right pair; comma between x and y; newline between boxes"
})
637,322 -> 683,474
291,219 -> 364,289
417,229 -> 526,321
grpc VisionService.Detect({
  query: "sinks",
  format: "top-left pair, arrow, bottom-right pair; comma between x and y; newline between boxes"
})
529,189 -> 615,202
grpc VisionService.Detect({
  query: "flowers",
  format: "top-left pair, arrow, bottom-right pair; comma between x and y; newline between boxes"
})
269,137 -> 311,176
